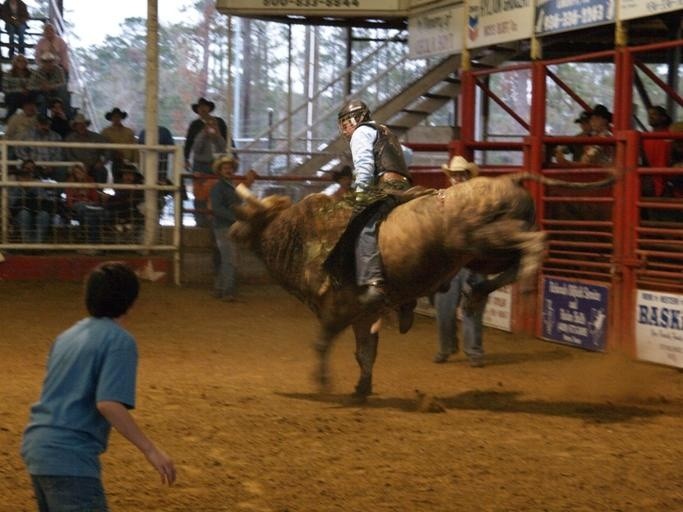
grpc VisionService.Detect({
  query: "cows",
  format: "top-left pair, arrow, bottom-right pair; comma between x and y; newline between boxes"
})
224,172 -> 617,400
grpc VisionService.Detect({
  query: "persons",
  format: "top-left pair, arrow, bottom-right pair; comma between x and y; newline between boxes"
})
191,117 -> 235,257
431,156 -> 489,368
208,155 -> 247,304
550,112 -> 594,164
183,97 -> 238,229
0,99 -> 175,244
337,100 -> 418,334
330,167 -> 356,200
0,1 -> 29,59
190,156 -> 258,276
0,23 -> 70,119
642,103 -> 683,280
20,261 -> 176,511
555,103 -> 617,281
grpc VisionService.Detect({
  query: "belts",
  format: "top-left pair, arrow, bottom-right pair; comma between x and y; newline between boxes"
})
377,172 -> 410,185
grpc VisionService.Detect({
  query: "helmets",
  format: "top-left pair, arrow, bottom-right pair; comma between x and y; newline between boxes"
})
335,99 -> 369,140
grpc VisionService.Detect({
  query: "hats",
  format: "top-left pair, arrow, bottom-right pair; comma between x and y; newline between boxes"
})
574,104 -> 613,124
105,107 -> 127,121
40,52 -> 55,61
211,156 -> 239,173
442,155 -> 480,184
333,166 -> 352,181
192,97 -> 214,113
69,114 -> 91,129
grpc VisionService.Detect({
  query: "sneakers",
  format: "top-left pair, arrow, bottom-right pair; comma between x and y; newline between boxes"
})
358,287 -> 386,305
432,347 -> 458,363
468,357 -> 483,367
400,299 -> 417,333
